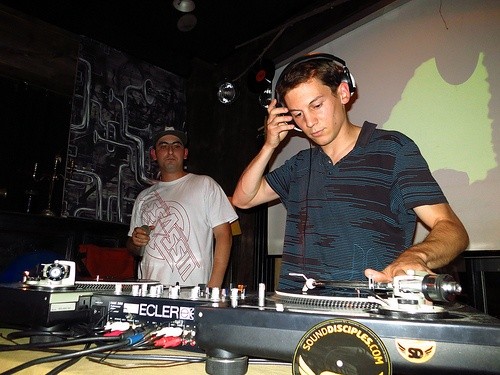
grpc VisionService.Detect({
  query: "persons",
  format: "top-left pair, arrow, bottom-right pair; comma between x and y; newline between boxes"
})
128,126 -> 238,290
232,53 -> 470,296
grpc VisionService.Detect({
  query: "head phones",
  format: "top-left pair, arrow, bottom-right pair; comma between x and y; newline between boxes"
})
273,52 -> 356,133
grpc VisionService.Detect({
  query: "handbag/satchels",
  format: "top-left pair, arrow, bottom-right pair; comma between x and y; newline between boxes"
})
76,243 -> 136,281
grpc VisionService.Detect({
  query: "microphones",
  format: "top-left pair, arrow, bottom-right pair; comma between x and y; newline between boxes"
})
137,225 -> 150,262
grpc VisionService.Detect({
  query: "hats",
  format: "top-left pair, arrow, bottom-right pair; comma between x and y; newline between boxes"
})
154,126 -> 187,150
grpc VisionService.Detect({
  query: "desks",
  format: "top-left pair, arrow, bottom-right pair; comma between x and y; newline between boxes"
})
0,328 -> 293,375
